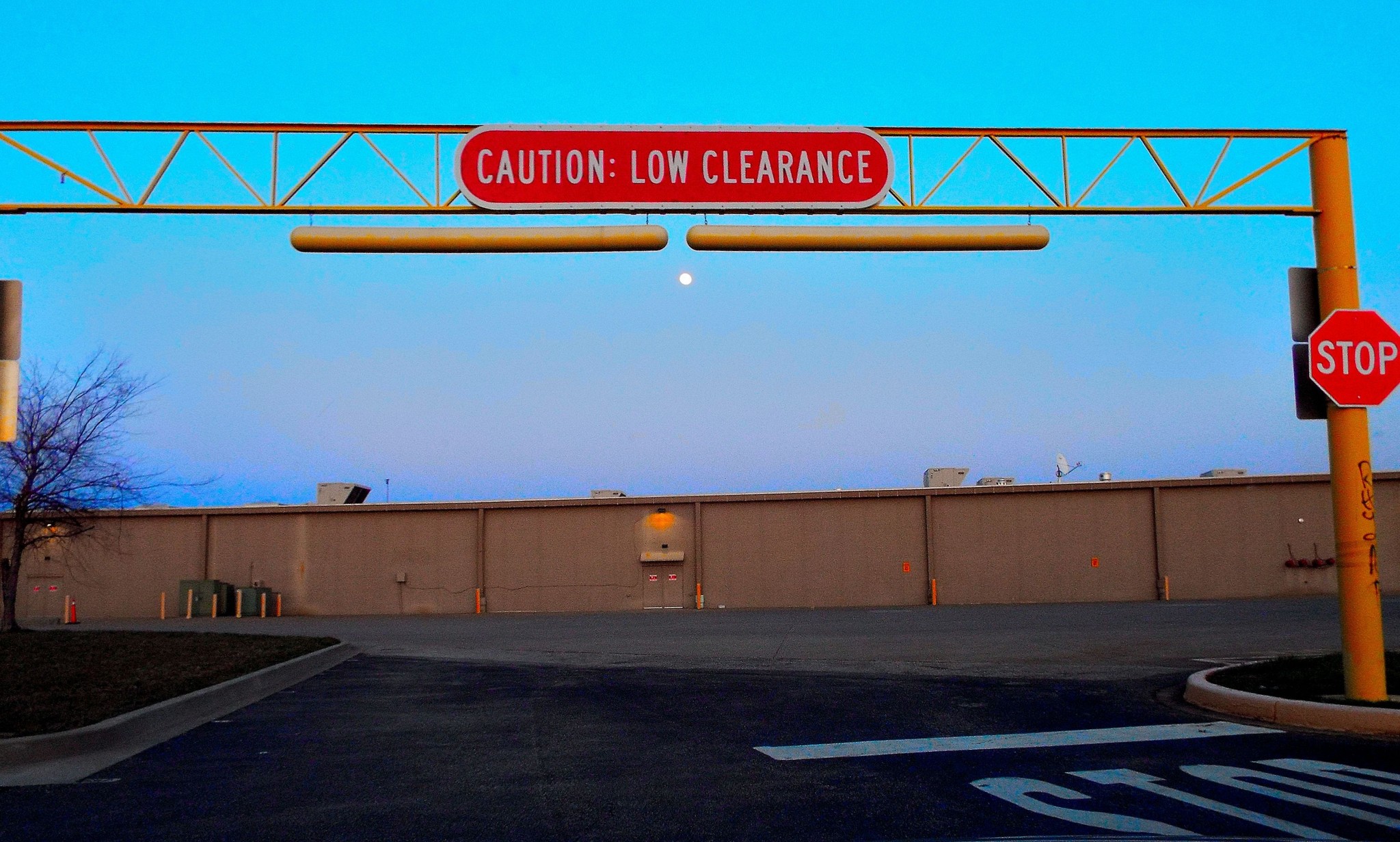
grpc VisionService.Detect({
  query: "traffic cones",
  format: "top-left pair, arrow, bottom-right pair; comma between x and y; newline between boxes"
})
66,599 -> 81,624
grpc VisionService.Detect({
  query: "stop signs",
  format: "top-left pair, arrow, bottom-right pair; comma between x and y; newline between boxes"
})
1308,311 -> 1400,406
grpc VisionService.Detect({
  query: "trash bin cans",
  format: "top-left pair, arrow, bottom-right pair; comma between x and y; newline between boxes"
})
199,580 -> 219,616
179,580 -> 200,615
239,587 -> 272,616
214,584 -> 234,616
